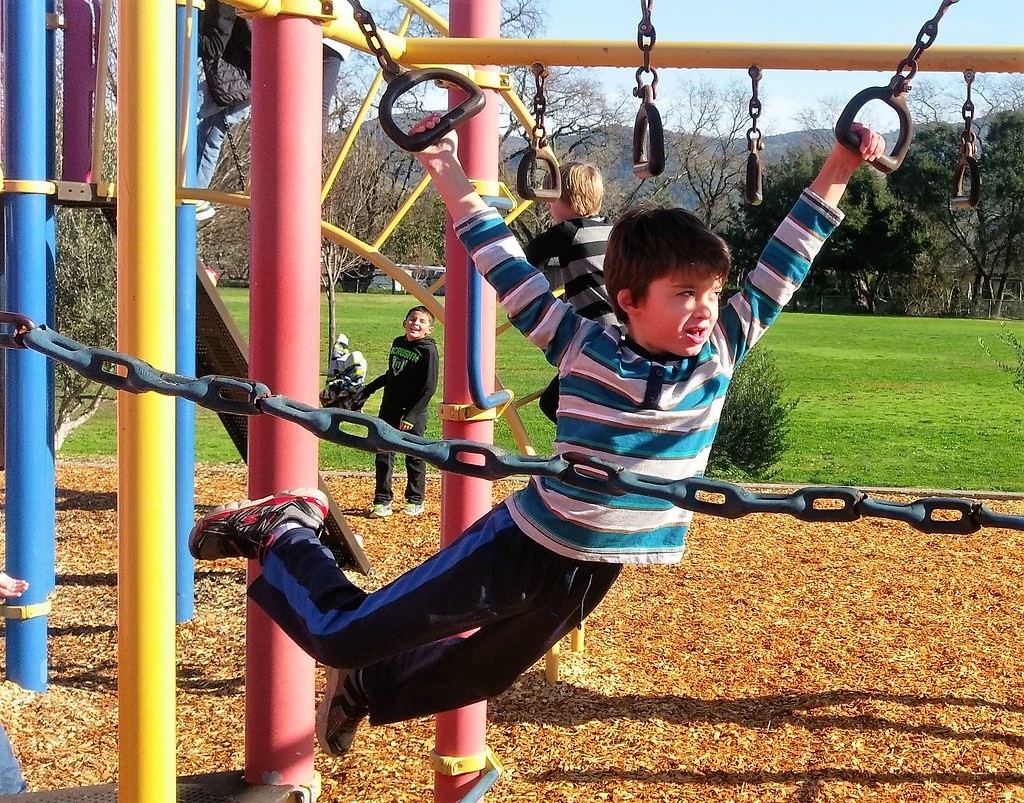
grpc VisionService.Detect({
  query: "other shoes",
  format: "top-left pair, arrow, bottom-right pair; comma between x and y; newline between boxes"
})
182,200 -> 215,221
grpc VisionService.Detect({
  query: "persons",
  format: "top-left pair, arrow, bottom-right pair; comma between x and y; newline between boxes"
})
183,0 -> 255,221
188,112 -> 886,757
357,305 -> 439,517
0,570 -> 29,797
518,161 -> 617,428
321,1 -> 364,155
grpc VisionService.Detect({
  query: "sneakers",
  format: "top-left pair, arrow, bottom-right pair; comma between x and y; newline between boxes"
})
187,487 -> 329,561
370,503 -> 392,518
402,502 -> 424,516
316,666 -> 368,757
